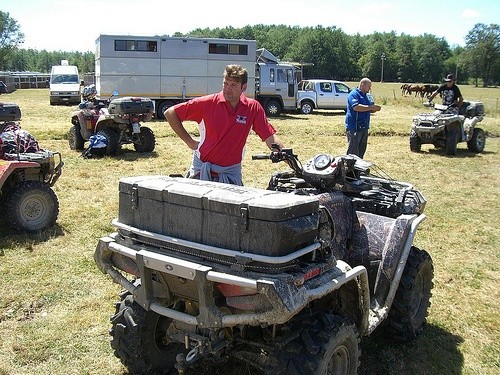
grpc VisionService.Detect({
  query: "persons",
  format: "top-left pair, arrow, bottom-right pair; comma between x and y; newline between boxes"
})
345,78 -> 382,159
163,64 -> 288,186
427,73 -> 464,115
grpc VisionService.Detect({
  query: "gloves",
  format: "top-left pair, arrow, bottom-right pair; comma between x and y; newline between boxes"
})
453,107 -> 458,112
426,101 -> 430,106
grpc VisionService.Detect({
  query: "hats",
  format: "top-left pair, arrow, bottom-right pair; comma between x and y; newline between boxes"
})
444,74 -> 455,81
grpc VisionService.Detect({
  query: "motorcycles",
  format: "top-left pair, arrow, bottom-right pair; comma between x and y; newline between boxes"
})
91,143 -> 436,375
0,101 -> 65,234
68,84 -> 157,160
409,100 -> 486,156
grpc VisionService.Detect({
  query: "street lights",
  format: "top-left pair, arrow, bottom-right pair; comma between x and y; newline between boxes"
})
381,53 -> 387,83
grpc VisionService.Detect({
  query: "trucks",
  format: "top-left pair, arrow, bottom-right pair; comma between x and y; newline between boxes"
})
93,33 -> 300,121
45,60 -> 85,105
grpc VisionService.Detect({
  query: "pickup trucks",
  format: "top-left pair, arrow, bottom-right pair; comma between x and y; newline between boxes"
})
298,79 -> 375,114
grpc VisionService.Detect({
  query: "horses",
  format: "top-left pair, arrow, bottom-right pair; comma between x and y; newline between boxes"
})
401,83 -> 440,99
